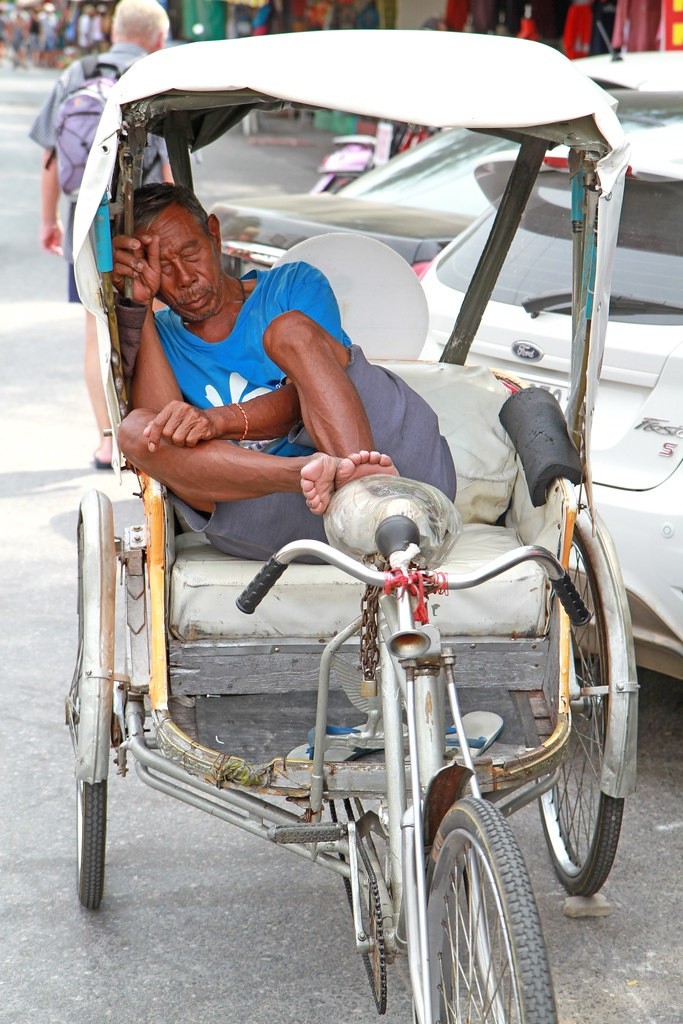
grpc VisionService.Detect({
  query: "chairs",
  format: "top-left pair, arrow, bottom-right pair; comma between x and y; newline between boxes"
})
155,359 -> 563,644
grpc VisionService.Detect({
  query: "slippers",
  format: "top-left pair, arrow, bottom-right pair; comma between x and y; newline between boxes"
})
287,718 -> 408,761
404,710 -> 504,762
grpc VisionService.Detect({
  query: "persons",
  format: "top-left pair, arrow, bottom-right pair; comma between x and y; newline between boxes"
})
27,0 -> 204,470
418,17 -> 447,31
1,0 -> 113,71
111,182 -> 459,566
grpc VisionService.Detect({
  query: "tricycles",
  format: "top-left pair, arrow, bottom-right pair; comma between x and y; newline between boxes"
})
60,24 -> 643,1022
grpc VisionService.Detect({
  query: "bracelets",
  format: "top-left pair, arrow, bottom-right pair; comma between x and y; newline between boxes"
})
231,403 -> 249,442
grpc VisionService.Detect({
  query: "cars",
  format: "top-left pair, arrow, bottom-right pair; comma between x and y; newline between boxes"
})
329,40 -> 683,219
395,116 -> 683,694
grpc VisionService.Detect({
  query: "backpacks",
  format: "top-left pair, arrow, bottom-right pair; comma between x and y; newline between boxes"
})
53,53 -> 160,204
